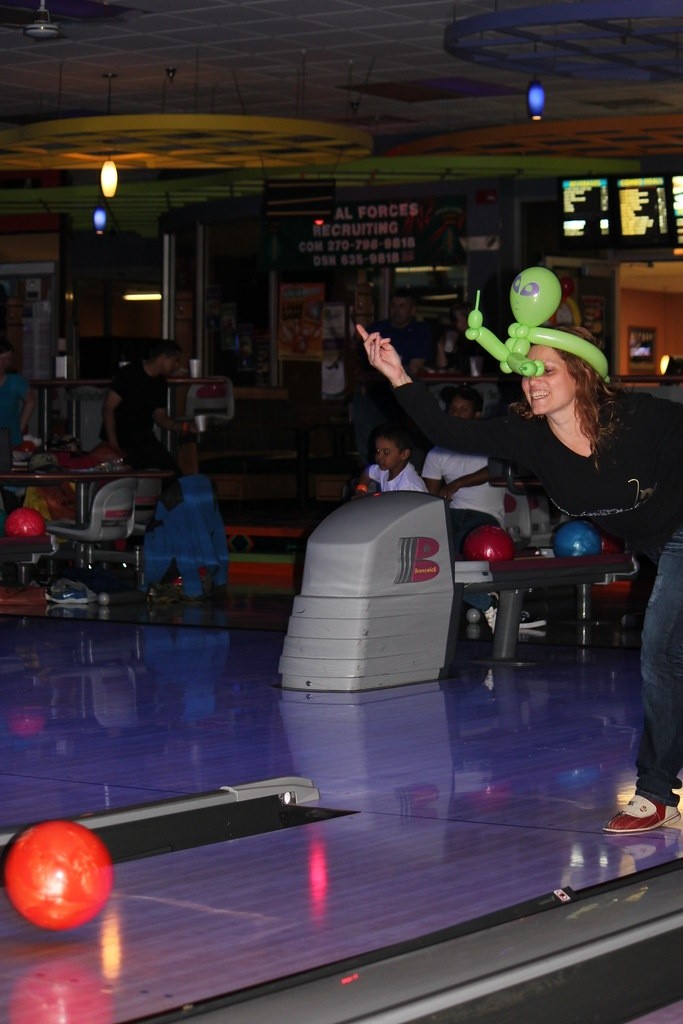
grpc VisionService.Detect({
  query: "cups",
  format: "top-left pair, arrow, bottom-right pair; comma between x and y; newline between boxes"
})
194,414 -> 207,433
469,356 -> 483,377
189,358 -> 203,379
444,330 -> 459,353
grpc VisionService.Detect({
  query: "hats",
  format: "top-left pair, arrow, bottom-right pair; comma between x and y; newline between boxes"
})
439,386 -> 484,410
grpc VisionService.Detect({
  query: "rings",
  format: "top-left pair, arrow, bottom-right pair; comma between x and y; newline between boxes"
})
444,495 -> 447,499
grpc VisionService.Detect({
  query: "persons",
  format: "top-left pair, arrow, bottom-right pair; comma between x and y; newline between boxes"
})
432,300 -> 486,378
358,420 -> 430,496
99,336 -> 198,495
351,286 -> 432,394
356,320 -> 683,833
417,386 -> 509,631
46,536 -> 115,603
0,342 -> 36,448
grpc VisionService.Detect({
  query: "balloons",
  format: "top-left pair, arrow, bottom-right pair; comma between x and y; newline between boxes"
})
465,266 -> 613,385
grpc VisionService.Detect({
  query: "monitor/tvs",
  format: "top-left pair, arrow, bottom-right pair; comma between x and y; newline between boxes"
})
557,173 -> 682,249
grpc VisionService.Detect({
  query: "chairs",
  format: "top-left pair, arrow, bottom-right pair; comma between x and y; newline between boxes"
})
0,478 -> 162,602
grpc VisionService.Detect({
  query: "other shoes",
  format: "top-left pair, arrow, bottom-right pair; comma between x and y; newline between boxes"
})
482,593 -> 500,634
519,610 -> 546,630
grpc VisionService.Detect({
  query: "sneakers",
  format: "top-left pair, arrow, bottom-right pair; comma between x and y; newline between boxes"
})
44,586 -> 89,604
601,794 -> 680,832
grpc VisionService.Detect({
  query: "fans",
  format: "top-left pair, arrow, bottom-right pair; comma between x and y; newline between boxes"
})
0,0 -> 120,38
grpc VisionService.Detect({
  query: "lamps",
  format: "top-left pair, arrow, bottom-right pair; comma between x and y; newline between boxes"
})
526,26 -> 545,121
93,73 -> 118,233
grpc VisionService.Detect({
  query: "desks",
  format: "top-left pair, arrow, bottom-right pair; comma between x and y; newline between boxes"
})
0,469 -> 175,586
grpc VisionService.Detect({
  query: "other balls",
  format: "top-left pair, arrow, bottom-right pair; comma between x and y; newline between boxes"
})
552,520 -> 603,558
2,820 -> 116,931
462,525 -> 515,564
4,506 -> 47,537
